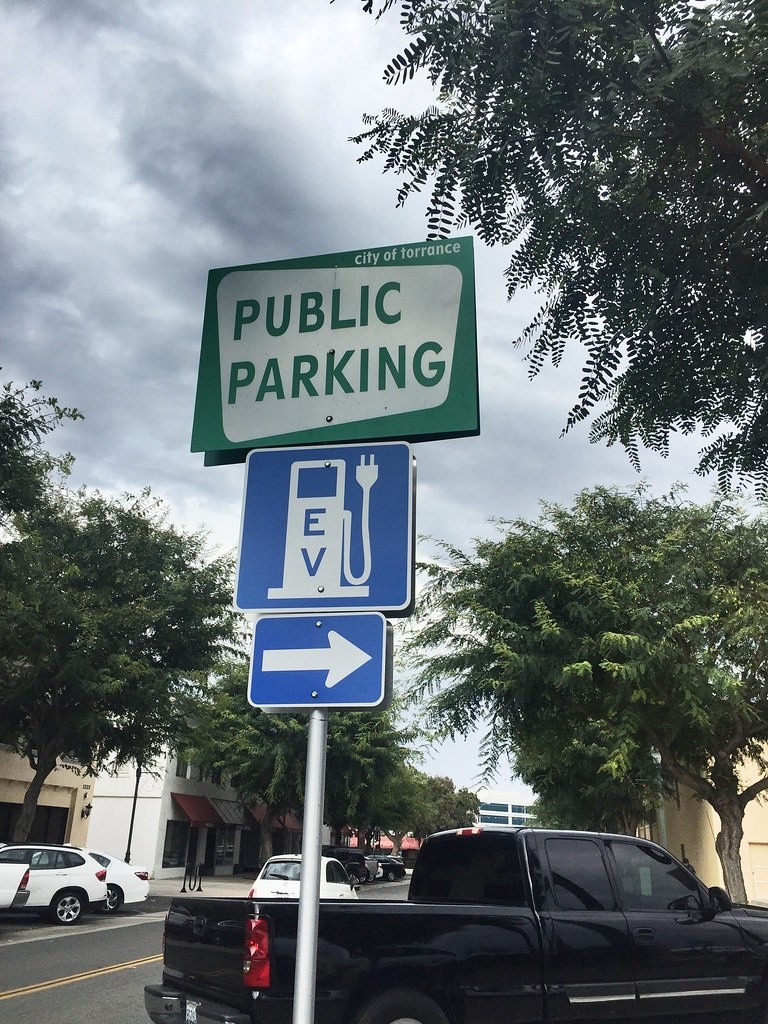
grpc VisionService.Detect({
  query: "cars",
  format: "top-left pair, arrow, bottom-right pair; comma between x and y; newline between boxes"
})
29,843 -> 150,915
364,853 -> 407,883
245,852 -> 360,900
0,841 -> 108,926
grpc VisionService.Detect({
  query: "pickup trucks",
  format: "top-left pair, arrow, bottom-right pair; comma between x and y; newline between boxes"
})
144,826 -> 768,1024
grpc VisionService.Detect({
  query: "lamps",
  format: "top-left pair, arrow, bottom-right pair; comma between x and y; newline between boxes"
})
81,802 -> 93,819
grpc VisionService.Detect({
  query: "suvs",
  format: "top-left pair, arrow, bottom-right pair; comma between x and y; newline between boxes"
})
320,843 -> 369,885
